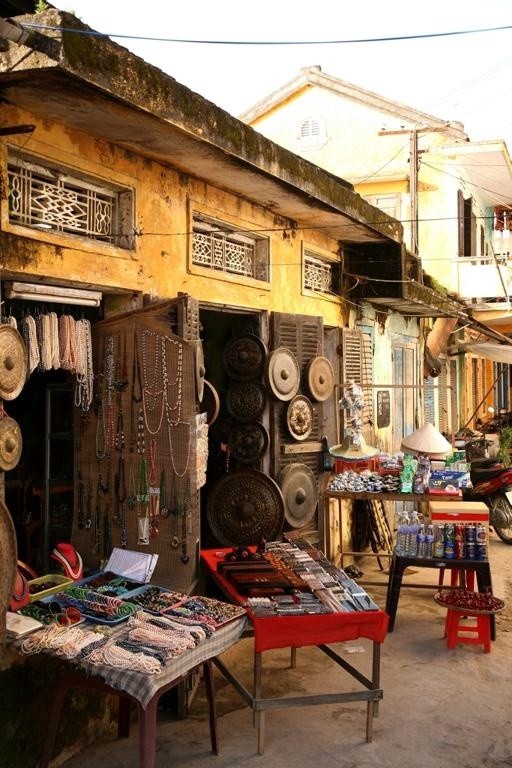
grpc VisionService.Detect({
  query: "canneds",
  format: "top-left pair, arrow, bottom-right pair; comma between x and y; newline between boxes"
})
434,522 -> 487,560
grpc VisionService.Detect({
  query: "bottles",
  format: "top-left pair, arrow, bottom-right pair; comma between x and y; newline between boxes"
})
395,510 -> 435,559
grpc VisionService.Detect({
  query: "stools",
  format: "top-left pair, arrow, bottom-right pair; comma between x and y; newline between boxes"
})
443,608 -> 491,653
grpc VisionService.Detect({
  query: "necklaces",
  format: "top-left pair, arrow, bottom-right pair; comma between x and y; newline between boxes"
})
1,312 -> 218,676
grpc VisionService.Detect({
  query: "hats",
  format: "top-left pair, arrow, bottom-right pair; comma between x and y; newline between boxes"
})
399,422 -> 452,458
328,431 -> 380,460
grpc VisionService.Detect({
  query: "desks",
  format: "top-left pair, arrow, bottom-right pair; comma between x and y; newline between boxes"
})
6,566 -> 248,767
201,539 -> 390,755
386,551 -> 496,642
323,487 -> 465,592
